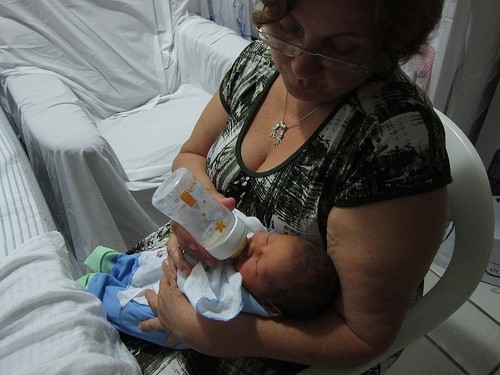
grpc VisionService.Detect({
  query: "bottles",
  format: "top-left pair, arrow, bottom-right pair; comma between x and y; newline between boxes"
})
151,168 -> 247,261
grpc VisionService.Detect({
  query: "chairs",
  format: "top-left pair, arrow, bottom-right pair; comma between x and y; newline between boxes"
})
0,0 -> 250,254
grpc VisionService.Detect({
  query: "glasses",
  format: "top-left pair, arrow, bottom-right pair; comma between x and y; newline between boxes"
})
255,4 -> 381,82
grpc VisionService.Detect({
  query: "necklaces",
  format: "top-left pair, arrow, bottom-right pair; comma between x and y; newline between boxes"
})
269,89 -> 323,147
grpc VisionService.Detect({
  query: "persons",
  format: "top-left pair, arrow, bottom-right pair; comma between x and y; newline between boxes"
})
73,212 -> 342,350
120,0 -> 454,375
